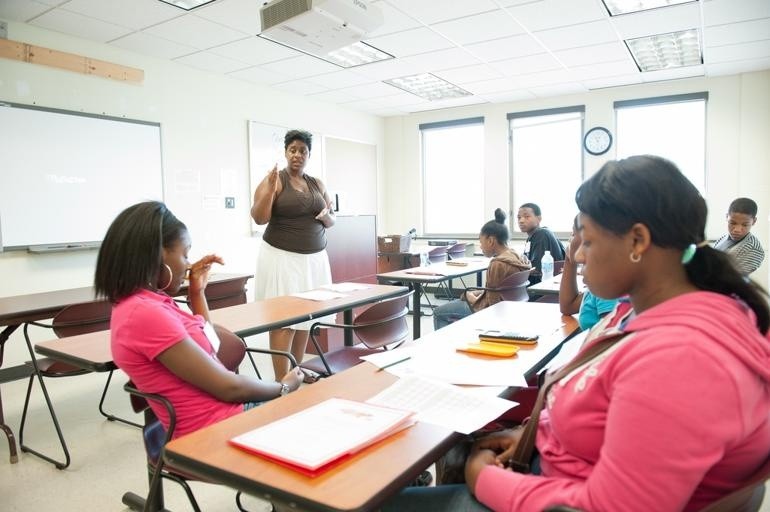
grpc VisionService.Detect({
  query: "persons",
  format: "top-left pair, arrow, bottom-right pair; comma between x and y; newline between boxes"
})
388,154 -> 770,512
714,198 -> 765,277
433,209 -> 531,330
250,128 -> 336,383
518,203 -> 566,302
558,215 -> 620,333
94,201 -> 304,442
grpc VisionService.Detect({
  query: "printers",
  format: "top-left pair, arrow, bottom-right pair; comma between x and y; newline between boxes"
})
255,0 -> 383,62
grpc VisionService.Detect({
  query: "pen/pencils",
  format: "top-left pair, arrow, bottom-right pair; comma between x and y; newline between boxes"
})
378,356 -> 411,372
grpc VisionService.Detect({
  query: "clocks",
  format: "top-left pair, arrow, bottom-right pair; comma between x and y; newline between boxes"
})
584,127 -> 613,155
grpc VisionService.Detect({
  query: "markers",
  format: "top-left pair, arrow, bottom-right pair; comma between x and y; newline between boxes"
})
48,246 -> 65,248
67,245 -> 82,248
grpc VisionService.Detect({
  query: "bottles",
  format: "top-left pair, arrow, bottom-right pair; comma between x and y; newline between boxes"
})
540,250 -> 554,286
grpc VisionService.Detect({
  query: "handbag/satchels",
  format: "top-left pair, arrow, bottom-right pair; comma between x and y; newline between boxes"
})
437,430 -> 509,485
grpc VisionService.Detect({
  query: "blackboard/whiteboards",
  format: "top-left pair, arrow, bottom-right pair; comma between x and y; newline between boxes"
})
0,99 -> 165,255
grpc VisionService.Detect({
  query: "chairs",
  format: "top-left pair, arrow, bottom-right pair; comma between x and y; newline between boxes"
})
125,341 -> 302,512
23,304 -> 155,471
540,258 -> 567,276
297,289 -> 417,376
462,266 -> 537,315
428,243 -> 474,265
692,461 -> 770,512
189,273 -> 265,382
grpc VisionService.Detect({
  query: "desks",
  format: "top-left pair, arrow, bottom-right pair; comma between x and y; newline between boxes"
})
160,299 -> 591,511
375,255 -> 491,340
34,281 -> 409,433
525,263 -> 592,298
0,272 -> 218,464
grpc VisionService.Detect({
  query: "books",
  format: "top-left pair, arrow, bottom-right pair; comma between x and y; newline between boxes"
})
228,398 -> 420,479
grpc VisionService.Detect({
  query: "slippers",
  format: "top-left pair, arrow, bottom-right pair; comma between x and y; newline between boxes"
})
302,373 -> 315,384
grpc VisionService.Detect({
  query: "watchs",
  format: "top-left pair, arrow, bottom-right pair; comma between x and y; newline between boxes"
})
279,383 -> 289,397
